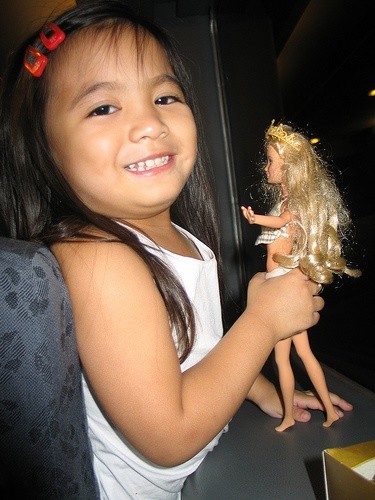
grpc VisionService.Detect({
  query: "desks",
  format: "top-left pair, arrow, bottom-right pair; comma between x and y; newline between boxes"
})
181,346 -> 375,500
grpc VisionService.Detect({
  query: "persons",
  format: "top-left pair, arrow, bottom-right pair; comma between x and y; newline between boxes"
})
0,2 -> 326,499
242,119 -> 362,432
